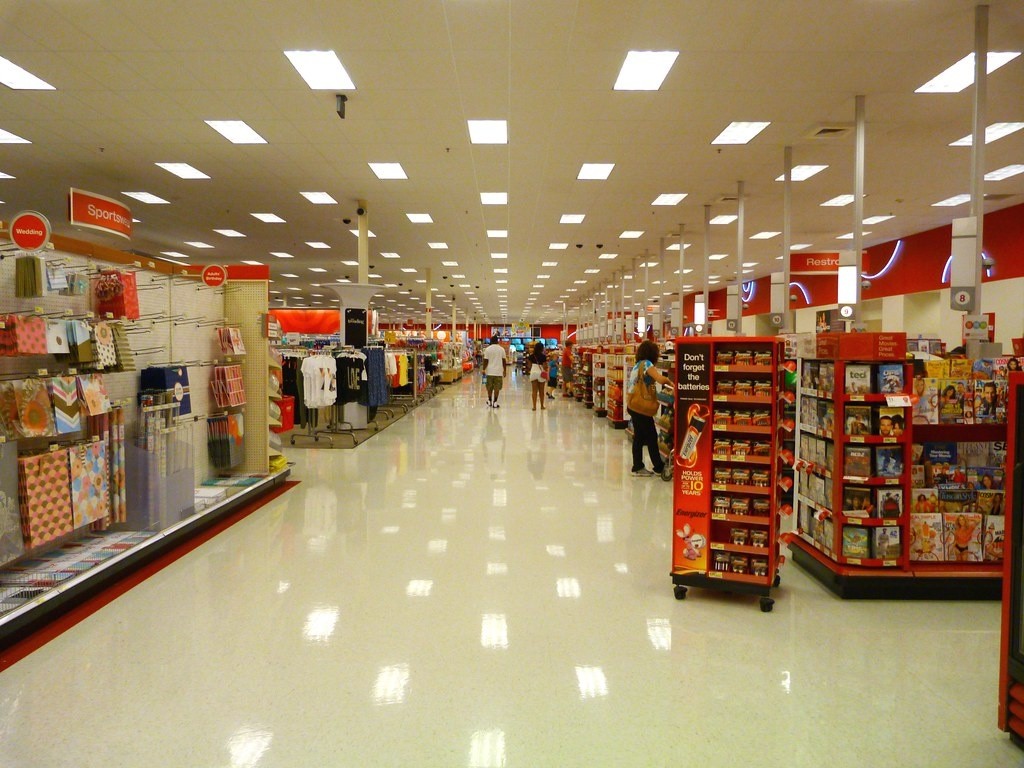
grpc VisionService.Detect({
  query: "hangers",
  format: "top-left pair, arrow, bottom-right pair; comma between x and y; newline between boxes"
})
385,349 -> 407,355
363,342 -> 383,350
278,348 -> 332,361
336,350 -> 358,359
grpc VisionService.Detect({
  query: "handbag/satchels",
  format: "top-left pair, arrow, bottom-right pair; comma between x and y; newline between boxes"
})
140,366 -> 192,415
626,360 -> 660,417
1,308 -> 136,550
540,371 -> 548,378
207,325 -> 246,469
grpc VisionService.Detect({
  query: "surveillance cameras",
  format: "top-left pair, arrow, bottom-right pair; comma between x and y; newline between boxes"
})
345,276 -> 349,279
450,285 -> 454,287
476,286 -> 479,288
683,315 -> 688,320
369,266 -> 374,269
708,311 -> 713,316
357,208 -> 365,215
743,304 -> 749,309
596,244 -> 603,248
983,258 -> 994,269
443,276 -> 447,279
791,295 -> 797,302
399,284 -> 403,286
344,220 -> 352,224
862,281 -> 871,290
576,244 -> 583,248
409,289 -> 412,292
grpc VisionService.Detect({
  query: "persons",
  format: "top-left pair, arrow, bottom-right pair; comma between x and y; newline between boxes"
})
912,356 -> 1024,424
548,354 -> 560,399
503,330 -> 511,337
561,340 -> 576,398
842,361 -> 905,560
482,335 -> 507,408
527,343 -> 550,410
626,340 -> 675,477
911,442 -> 1007,561
493,327 -> 501,336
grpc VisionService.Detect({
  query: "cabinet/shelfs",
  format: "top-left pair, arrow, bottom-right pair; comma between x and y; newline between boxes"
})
439,342 -> 464,384
268,336 -> 282,458
669,335 -> 785,612
913,425 -> 1006,602
604,354 -> 629,430
791,356 -> 916,601
592,353 -> 607,418
580,348 -> 594,409
572,360 -> 583,402
783,355 -> 797,499
624,361 -> 675,464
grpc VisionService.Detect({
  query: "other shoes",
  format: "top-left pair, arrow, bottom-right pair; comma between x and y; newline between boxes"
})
545,392 -> 550,396
652,467 -> 663,475
548,395 -> 555,399
563,393 -> 569,397
569,392 -> 573,396
631,467 -> 654,476
486,400 -> 492,406
493,401 -> 500,408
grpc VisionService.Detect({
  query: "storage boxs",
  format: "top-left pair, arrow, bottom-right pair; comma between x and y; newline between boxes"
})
796,333 -> 816,357
814,332 -> 906,359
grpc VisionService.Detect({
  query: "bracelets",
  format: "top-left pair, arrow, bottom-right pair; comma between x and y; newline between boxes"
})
670,381 -> 673,386
482,370 -> 486,373
